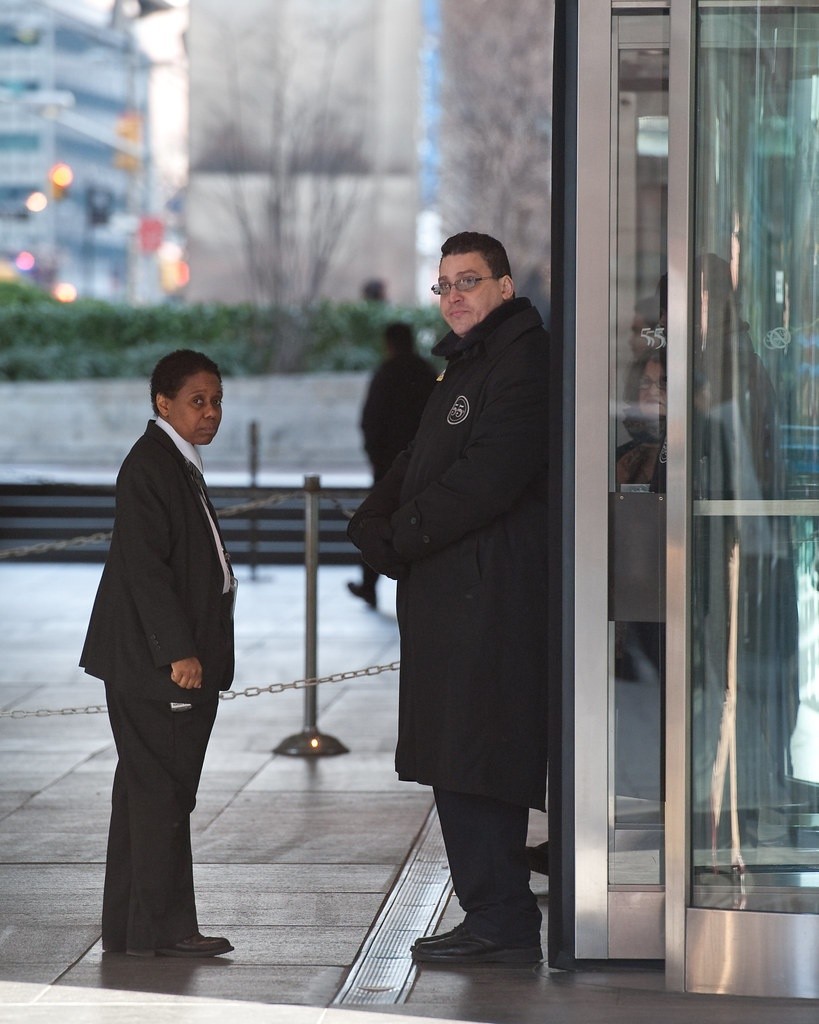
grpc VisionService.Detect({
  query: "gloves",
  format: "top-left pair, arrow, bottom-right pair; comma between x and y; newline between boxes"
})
348,511 -> 409,580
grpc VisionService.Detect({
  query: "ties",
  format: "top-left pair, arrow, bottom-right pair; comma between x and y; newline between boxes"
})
188,462 -> 208,505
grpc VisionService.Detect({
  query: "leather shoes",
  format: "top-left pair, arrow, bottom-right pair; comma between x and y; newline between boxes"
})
126,933 -> 234,958
410,924 -> 543,965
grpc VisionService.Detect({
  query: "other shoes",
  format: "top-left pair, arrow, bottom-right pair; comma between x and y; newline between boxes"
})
348,582 -> 377,606
525,842 -> 550,875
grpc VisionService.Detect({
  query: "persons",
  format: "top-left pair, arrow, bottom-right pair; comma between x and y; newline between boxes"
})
348,322 -> 441,607
346,231 -> 548,962
611,253 -> 802,853
79,349 -> 235,960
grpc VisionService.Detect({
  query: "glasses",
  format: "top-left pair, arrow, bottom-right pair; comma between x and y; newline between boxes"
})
633,327 -> 650,336
636,374 -> 666,388
431,276 -> 499,295
